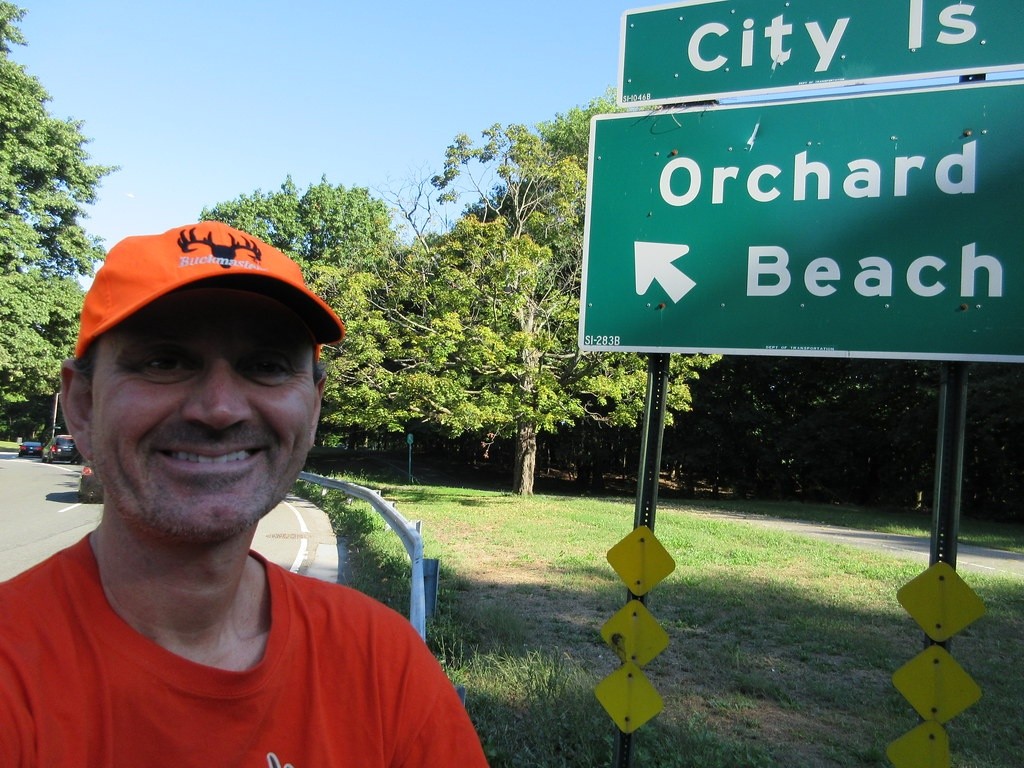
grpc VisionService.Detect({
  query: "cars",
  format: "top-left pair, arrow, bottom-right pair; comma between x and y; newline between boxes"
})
79,462 -> 104,502
18,442 -> 43,457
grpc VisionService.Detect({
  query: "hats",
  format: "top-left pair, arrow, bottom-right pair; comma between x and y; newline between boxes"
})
75,221 -> 345,364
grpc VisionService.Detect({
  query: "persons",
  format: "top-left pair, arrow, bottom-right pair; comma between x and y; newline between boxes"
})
0,221 -> 494,768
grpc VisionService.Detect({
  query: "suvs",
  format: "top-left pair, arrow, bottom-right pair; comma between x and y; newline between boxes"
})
42,435 -> 83,465
